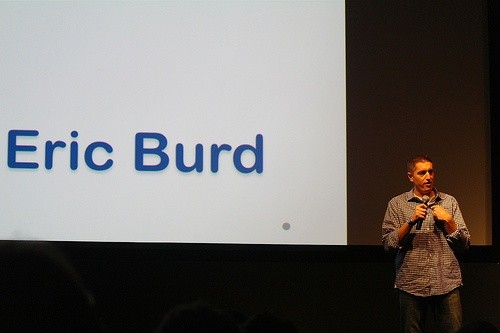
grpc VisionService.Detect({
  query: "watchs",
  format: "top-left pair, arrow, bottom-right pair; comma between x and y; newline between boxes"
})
407,217 -> 413,228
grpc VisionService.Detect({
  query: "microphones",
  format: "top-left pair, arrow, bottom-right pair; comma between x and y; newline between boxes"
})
416,195 -> 430,230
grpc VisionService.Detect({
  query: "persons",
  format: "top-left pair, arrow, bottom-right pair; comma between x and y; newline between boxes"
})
381,154 -> 472,333
